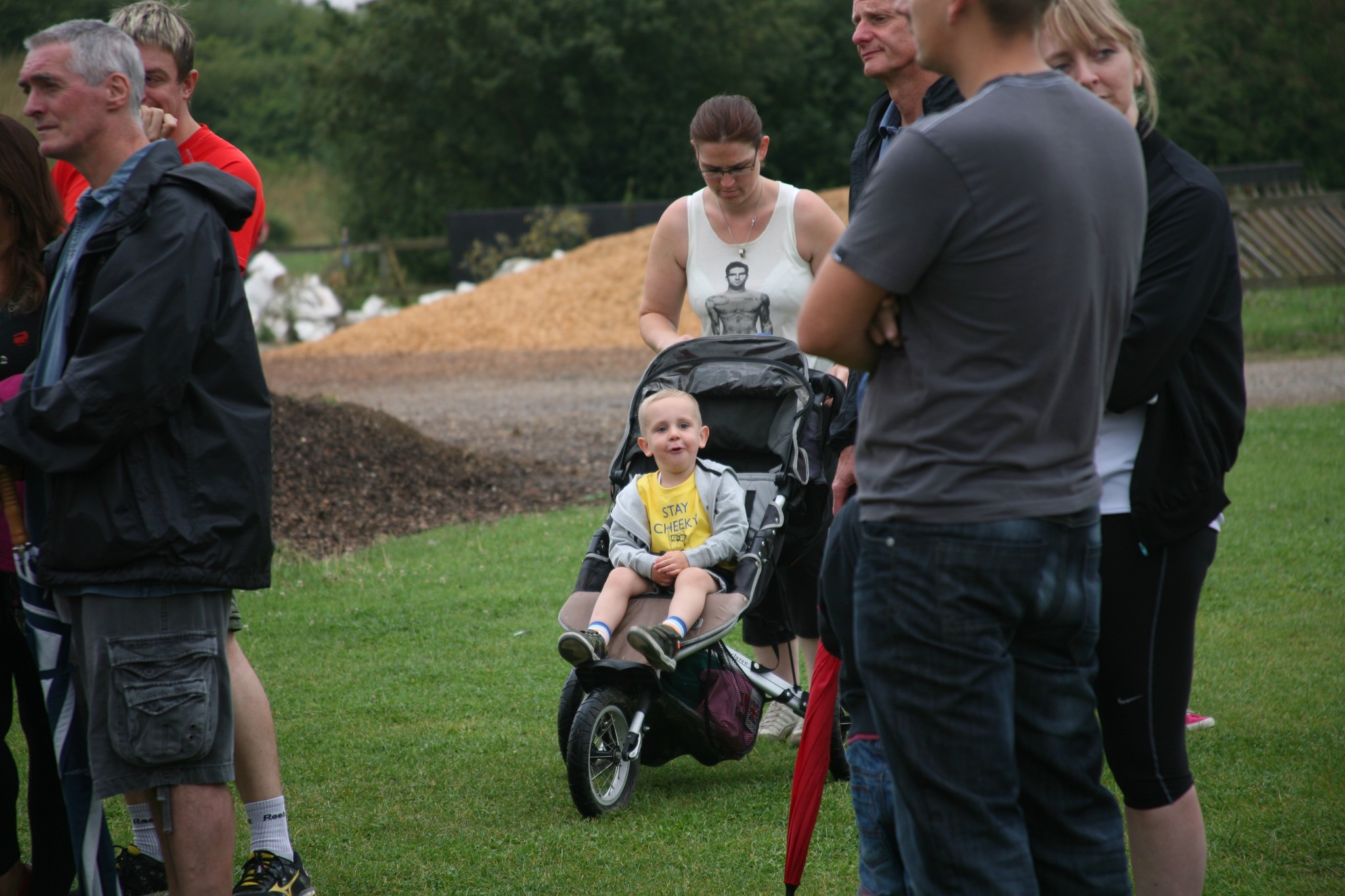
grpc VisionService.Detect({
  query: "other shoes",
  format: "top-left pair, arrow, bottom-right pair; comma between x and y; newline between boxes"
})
1188,710 -> 1211,725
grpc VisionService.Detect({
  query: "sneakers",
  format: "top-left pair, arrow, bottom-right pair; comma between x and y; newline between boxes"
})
232,846 -> 316,896
785,714 -> 805,746
627,622 -> 682,671
755,698 -> 802,739
73,843 -> 171,896
557,627 -> 609,667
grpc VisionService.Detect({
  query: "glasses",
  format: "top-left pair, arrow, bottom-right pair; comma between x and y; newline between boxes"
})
696,146 -> 760,178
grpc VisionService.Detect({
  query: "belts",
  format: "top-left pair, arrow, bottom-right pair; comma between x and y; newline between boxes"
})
874,517 -> 1101,541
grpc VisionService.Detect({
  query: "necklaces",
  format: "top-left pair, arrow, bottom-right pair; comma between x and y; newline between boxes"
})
715,176 -> 762,257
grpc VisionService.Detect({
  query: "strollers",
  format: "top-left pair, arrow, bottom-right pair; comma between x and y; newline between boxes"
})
556,333 -> 851,817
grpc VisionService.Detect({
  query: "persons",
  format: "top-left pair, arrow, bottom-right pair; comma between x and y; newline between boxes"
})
558,389 -> 750,672
558,1 -> 1247,896
795,0 -> 1146,896
1,20 -> 272,896
0,1 -> 318,896
706,262 -> 773,336
636,96 -> 847,745
